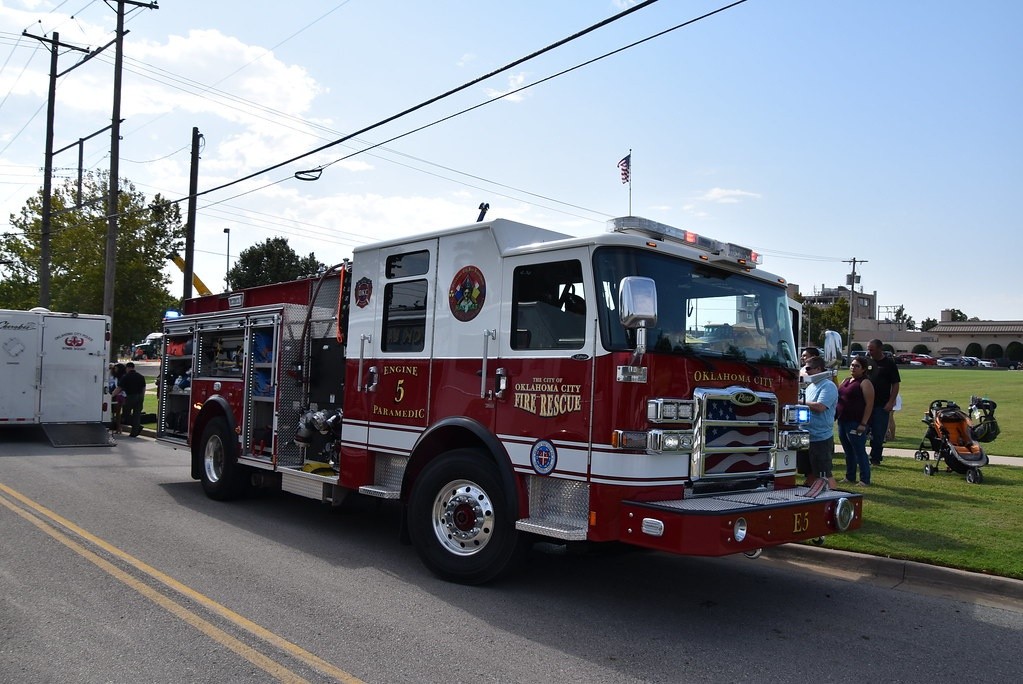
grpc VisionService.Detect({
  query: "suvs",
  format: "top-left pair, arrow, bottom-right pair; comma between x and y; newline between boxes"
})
849,351 -> 869,362
133,338 -> 160,358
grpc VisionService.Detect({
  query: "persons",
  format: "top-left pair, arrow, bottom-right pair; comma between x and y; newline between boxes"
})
865,340 -> 902,466
112,364 -> 127,434
121,350 -> 124,357
127,349 -> 131,355
884,393 -> 902,441
155,365 -> 161,419
796,356 -> 838,486
834,357 -> 875,486
111,362 -> 146,436
136,347 -> 143,361
799,348 -> 819,400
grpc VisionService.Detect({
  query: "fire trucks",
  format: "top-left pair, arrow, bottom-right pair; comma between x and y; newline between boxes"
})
156,215 -> 862,585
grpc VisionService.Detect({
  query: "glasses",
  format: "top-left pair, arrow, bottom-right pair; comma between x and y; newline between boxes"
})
806,367 -> 815,371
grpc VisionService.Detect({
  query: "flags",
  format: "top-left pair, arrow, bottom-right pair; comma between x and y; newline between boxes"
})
617,154 -> 630,184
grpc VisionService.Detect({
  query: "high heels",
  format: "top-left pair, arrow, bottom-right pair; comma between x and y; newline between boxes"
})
113,428 -> 122,434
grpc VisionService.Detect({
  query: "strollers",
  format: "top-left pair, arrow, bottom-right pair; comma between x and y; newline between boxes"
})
915,399 -> 989,483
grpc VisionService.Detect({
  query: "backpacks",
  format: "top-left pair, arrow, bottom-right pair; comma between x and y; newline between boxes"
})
970,402 -> 1000,443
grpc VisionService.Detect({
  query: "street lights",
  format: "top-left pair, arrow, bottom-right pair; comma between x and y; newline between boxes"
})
224,228 -> 230,291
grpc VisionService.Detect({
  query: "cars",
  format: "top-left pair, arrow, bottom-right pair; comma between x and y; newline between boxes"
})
882,351 -> 996,367
